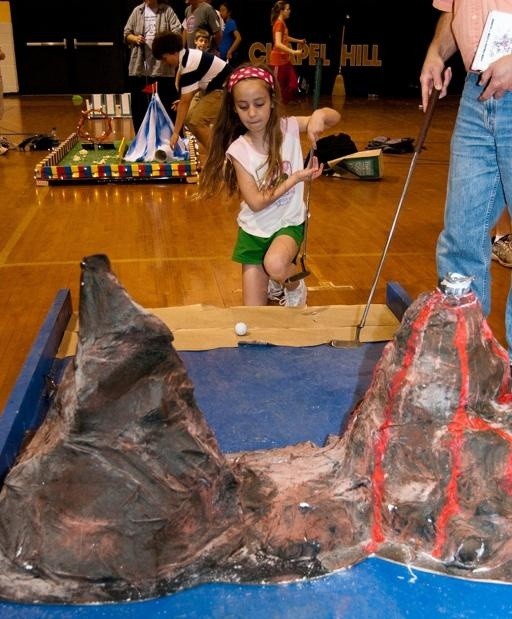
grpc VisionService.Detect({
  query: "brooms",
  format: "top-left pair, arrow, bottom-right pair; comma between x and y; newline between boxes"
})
332,25 -> 346,98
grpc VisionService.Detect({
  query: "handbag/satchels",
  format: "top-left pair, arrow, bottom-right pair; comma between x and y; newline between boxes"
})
327,148 -> 384,180
368,136 -> 415,154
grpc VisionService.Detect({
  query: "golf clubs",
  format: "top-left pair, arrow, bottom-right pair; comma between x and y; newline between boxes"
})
331,70 -> 444,349
284,148 -> 310,283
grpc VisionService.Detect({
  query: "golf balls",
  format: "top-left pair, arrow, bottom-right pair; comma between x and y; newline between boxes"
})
235,322 -> 247,336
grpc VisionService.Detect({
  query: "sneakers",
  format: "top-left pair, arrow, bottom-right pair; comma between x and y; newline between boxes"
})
491,235 -> 512,267
268,279 -> 283,301
285,279 -> 307,307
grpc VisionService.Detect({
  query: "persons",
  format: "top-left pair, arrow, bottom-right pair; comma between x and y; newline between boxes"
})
268,1 -> 306,117
192,63 -> 342,308
419,0 -> 511,365
122,0 -> 242,172
0,47 -> 19,156
491,206 -> 511,268
